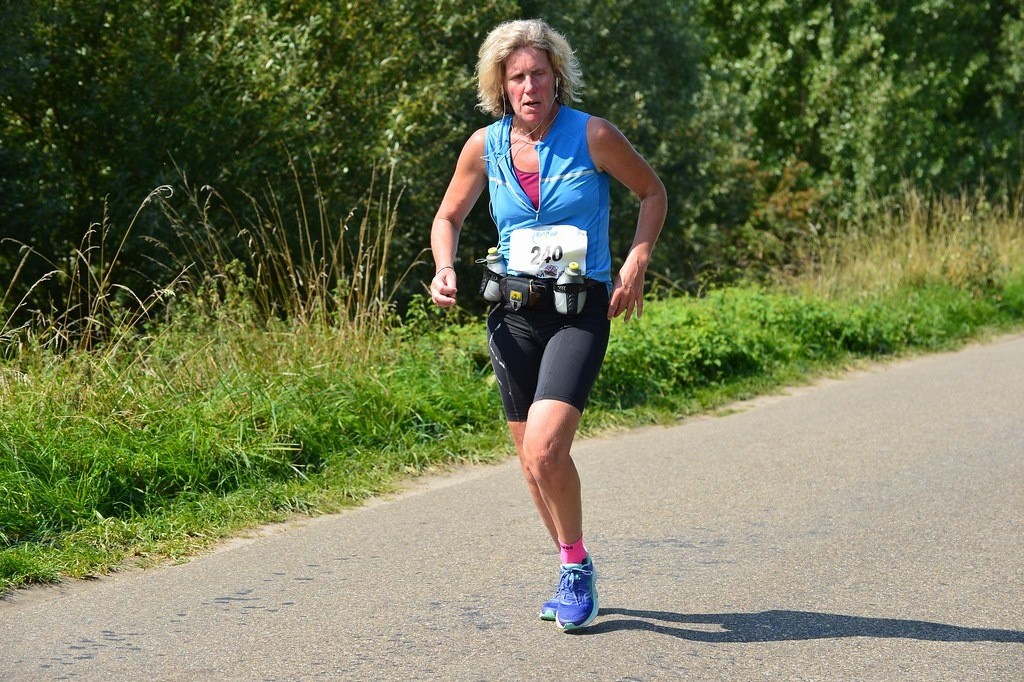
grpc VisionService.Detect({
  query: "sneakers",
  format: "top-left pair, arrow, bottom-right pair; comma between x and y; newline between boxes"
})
555,546 -> 599,631
539,591 -> 560,620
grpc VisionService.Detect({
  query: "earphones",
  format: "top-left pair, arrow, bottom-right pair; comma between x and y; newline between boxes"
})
555,75 -> 559,97
500,84 -> 504,94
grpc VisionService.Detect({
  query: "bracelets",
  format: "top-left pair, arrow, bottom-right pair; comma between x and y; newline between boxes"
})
436,265 -> 455,273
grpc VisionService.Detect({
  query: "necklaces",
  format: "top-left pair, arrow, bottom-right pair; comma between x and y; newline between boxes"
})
511,111 -> 561,144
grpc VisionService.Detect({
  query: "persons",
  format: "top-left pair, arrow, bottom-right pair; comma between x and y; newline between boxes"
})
431,19 -> 667,634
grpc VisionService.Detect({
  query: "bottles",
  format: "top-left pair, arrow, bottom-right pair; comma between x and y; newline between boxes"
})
475,247 -> 507,302
554,262 -> 586,314
